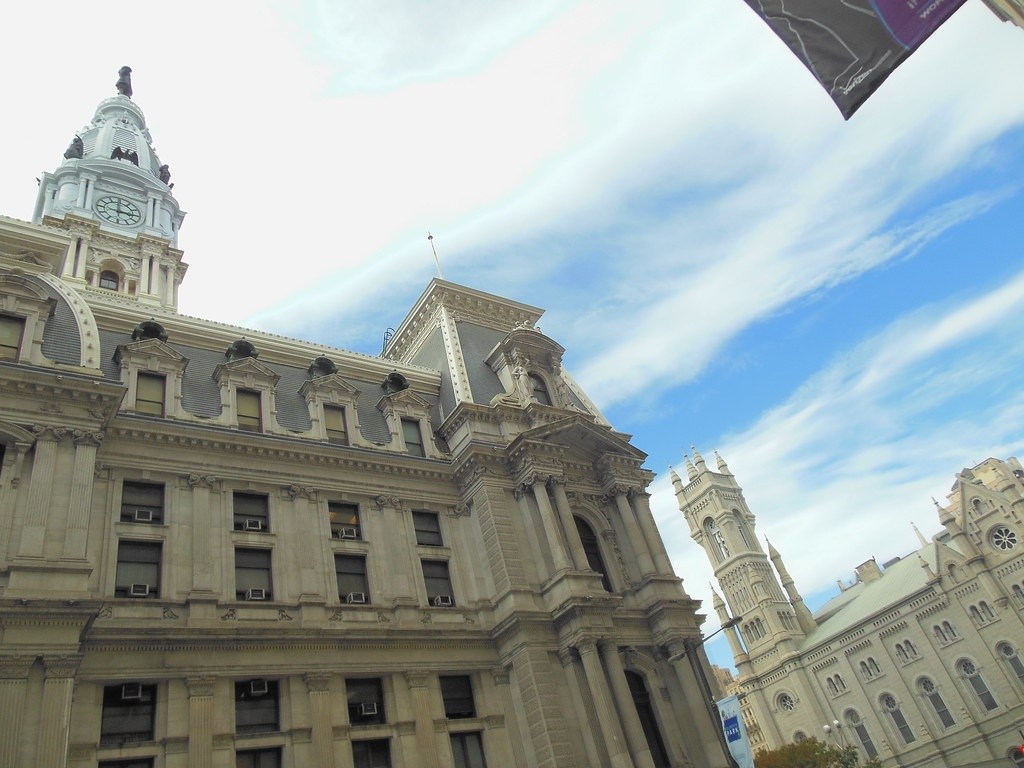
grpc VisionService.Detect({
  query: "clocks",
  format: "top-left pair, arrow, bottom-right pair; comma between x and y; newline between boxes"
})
96,196 -> 142,226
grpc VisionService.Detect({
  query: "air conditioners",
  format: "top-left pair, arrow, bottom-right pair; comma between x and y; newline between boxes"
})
243,520 -> 261,531
122,684 -> 142,698
246,588 -> 265,601
131,583 -> 150,597
347,592 -> 365,603
250,681 -> 267,694
339,527 -> 355,539
133,509 -> 153,523
435,595 -> 452,607
358,703 -> 377,717
